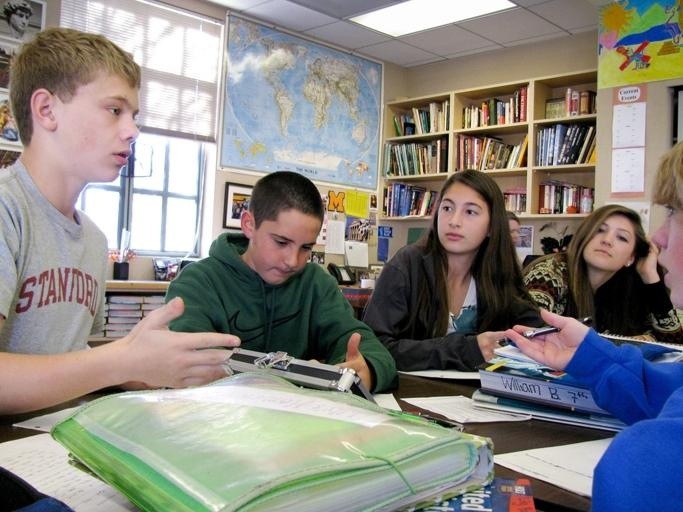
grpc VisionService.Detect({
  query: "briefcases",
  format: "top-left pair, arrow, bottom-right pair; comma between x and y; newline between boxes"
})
227,347 -> 377,405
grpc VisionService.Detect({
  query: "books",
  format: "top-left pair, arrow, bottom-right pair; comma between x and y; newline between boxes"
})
467,325 -> 683,433
91,288 -> 166,340
379,82 -> 596,220
48,369 -> 495,511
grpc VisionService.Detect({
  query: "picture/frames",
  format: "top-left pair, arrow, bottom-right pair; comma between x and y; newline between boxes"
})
669,82 -> 681,148
221,181 -> 256,230
0,0 -> 49,155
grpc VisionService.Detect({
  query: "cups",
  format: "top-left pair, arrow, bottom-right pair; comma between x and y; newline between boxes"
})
360,279 -> 375,289
112,262 -> 129,280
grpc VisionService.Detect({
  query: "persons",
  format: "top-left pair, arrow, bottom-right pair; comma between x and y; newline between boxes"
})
230,197 -> 249,220
357,170 -> 544,373
163,173 -> 399,399
522,205 -> 683,346
2,0 -> 36,41
504,209 -> 521,244
1,28 -> 243,419
505,139 -> 683,511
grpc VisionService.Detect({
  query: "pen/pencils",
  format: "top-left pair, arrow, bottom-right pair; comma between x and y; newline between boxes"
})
496,316 -> 592,346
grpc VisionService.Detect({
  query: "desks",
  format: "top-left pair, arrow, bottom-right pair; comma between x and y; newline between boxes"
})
0,364 -> 623,512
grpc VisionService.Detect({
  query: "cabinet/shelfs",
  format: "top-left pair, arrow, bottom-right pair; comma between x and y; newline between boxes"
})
88,278 -> 359,351
374,66 -> 598,222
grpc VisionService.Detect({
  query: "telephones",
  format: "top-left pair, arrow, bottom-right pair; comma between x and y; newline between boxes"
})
327,263 -> 356,285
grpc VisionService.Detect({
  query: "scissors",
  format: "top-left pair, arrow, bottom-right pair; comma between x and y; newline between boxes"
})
112,249 -> 136,263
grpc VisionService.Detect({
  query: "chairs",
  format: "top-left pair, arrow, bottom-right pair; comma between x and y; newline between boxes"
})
340,285 -> 373,321
